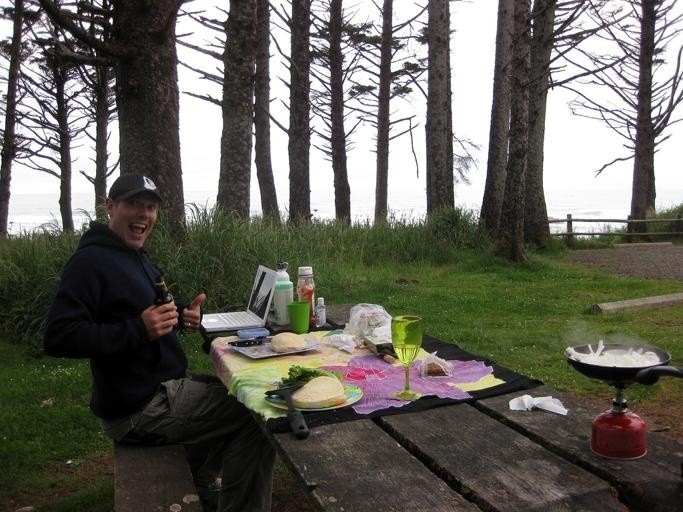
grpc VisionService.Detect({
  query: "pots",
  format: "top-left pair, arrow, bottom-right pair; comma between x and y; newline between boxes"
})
563,343 -> 683,387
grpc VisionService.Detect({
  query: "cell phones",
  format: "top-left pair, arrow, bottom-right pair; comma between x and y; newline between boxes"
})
236,327 -> 271,339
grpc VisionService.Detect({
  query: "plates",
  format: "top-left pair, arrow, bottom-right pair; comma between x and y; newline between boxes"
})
233,338 -> 321,360
269,377 -> 361,410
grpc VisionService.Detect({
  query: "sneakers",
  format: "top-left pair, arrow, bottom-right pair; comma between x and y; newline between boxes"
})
197,477 -> 222,493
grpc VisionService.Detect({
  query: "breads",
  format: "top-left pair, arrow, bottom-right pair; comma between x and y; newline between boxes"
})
270,331 -> 308,354
292,376 -> 347,408
424,361 -> 445,376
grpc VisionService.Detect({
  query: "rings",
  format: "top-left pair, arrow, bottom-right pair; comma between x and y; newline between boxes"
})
188,321 -> 190,328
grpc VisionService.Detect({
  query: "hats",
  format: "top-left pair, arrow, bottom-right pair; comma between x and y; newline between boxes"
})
109,174 -> 163,204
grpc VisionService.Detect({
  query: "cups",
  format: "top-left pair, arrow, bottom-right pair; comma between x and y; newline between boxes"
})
285,301 -> 310,333
314,297 -> 327,327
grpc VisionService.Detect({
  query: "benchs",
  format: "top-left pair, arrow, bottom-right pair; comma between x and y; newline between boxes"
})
112,441 -> 203,512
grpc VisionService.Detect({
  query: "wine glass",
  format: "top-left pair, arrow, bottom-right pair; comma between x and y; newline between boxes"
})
386,313 -> 426,402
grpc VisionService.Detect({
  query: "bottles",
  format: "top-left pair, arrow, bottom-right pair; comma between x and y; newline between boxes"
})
152,275 -> 178,333
274,280 -> 294,326
295,265 -> 315,325
273,260 -> 290,283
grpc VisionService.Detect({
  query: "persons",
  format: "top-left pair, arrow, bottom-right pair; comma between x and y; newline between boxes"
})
42,172 -> 277,511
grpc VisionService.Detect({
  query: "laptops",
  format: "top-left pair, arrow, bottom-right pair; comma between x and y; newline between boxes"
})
201,264 -> 280,333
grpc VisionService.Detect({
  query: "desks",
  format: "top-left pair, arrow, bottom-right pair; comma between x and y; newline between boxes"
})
201,304 -> 681,512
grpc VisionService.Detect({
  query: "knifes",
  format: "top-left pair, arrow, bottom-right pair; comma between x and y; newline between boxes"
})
227,336 -> 274,347
